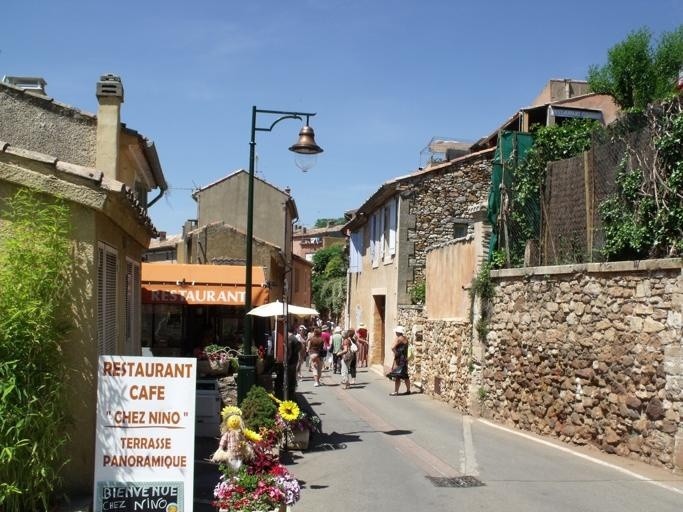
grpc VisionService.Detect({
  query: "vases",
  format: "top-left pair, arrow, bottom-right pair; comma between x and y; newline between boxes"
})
282,429 -> 309,450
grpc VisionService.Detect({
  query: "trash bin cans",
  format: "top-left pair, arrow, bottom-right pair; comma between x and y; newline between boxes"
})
195,377 -> 221,437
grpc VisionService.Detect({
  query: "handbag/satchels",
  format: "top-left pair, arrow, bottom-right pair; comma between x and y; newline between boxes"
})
320,349 -> 327,357
386,368 -> 402,380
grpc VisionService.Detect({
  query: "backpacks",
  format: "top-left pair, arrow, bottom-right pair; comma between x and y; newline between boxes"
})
343,339 -> 358,362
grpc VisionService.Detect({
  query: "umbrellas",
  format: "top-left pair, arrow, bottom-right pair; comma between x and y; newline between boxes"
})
246,298 -> 320,361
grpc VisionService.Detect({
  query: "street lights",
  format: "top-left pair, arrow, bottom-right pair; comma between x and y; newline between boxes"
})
234,105 -> 324,409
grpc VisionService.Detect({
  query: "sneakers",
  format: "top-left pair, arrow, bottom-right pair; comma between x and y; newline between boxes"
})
314,382 -> 324,387
404,391 -> 411,394
389,392 -> 398,396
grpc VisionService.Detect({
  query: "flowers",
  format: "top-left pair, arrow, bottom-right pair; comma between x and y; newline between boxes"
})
209,392 -> 300,512
276,412 -> 309,432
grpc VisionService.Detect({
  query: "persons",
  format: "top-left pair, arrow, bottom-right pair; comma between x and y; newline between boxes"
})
388,326 -> 411,396
272,315 -> 369,390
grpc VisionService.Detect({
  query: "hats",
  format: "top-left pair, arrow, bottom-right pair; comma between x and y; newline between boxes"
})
393,326 -> 406,334
321,325 -> 329,331
358,323 -> 366,328
335,326 -> 342,332
